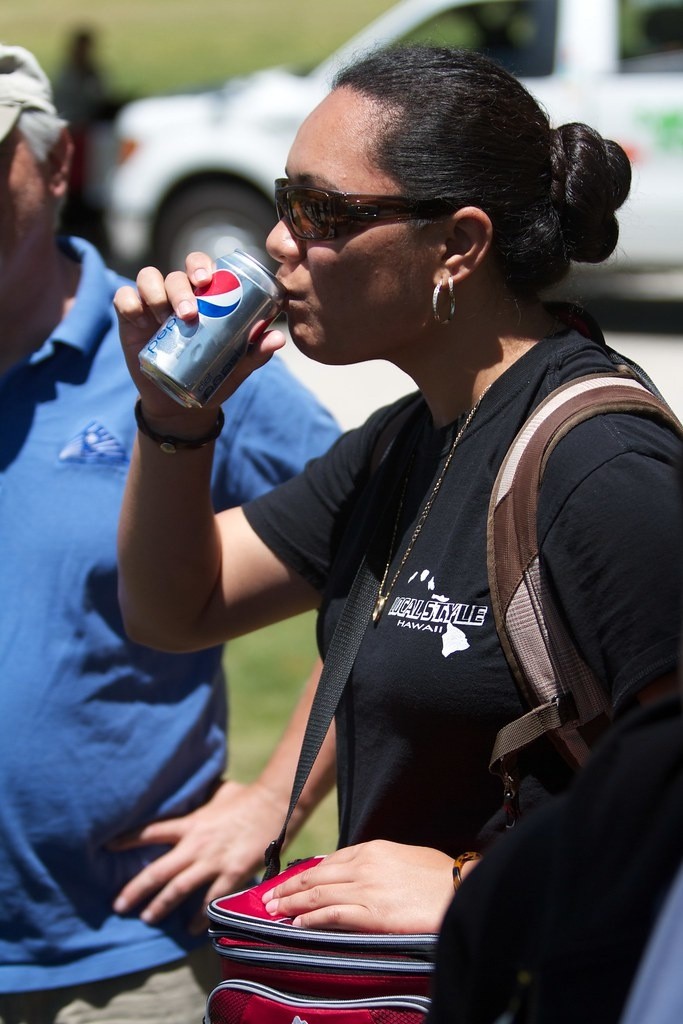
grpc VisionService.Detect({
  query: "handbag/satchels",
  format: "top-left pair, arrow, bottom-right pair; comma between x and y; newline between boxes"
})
208,849 -> 460,1023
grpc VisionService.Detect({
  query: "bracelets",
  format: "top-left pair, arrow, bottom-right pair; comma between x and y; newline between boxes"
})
454,850 -> 484,894
136,400 -> 228,455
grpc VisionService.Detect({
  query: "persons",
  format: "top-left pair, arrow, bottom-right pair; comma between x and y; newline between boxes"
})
0,51 -> 355,1024
120,43 -> 682,1024
48,29 -> 134,236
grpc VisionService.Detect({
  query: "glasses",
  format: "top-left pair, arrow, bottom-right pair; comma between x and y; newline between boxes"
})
273,178 -> 461,241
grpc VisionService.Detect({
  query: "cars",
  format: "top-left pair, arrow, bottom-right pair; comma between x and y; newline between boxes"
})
103,1 -> 683,327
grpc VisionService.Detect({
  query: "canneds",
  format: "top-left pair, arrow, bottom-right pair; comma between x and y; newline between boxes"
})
138,250 -> 289,408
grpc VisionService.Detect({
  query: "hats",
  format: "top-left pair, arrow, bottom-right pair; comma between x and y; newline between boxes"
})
0,45 -> 60,142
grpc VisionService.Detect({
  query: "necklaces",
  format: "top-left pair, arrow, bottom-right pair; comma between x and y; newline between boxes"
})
369,386 -> 492,627
